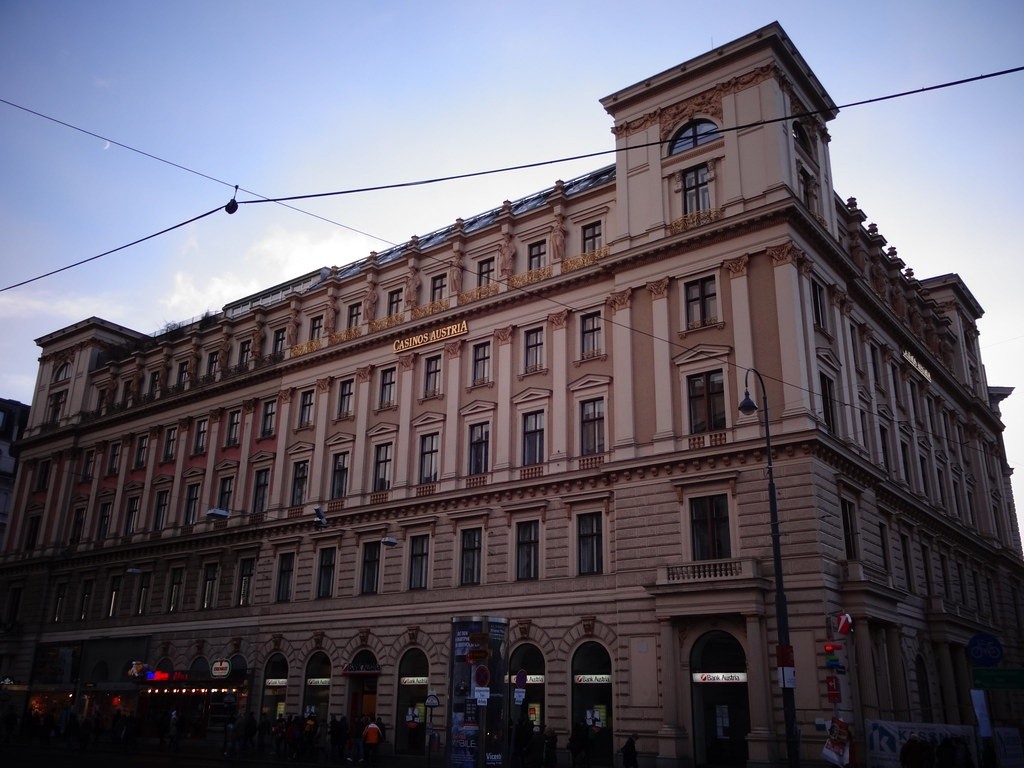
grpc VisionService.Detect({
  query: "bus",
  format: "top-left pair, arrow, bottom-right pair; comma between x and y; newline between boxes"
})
205,702 -> 227,731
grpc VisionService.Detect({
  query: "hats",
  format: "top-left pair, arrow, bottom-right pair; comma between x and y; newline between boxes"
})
533,726 -> 540,733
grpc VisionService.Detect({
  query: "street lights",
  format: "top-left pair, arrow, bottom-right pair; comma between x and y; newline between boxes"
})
737,365 -> 802,768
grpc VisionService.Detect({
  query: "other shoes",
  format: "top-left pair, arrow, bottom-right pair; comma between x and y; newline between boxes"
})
269,751 -> 276,755
293,753 -> 296,759
286,756 -> 291,761
347,758 -> 352,762
358,758 -> 364,762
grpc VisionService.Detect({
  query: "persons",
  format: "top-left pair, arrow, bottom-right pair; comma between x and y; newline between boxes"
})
223,709 -> 386,767
621,734 -> 639,768
525,726 -> 558,768
565,719 -> 613,768
900,734 -> 975,768
0,708 -> 185,753
978,738 -> 996,768
589,711 -> 598,721
